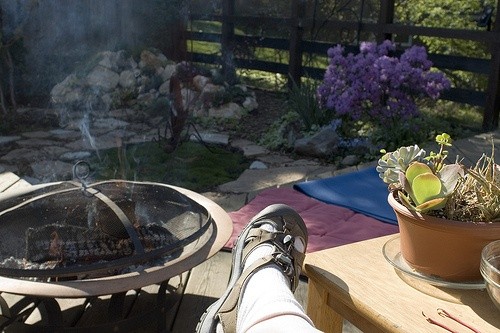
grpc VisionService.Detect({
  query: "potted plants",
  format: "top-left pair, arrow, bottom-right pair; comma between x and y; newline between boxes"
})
376,133 -> 500,290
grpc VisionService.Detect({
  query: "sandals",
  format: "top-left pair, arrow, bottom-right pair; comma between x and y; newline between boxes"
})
195,203 -> 308,332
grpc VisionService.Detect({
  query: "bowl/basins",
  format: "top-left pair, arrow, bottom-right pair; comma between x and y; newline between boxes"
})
479,240 -> 500,314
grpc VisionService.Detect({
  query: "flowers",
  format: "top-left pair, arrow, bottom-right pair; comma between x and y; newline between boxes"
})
317,40 -> 450,143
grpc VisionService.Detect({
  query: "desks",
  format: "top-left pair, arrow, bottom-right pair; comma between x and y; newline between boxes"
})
302,232 -> 500,333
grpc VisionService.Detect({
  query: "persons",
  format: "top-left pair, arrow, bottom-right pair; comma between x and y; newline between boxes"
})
196,204 -> 329,333
